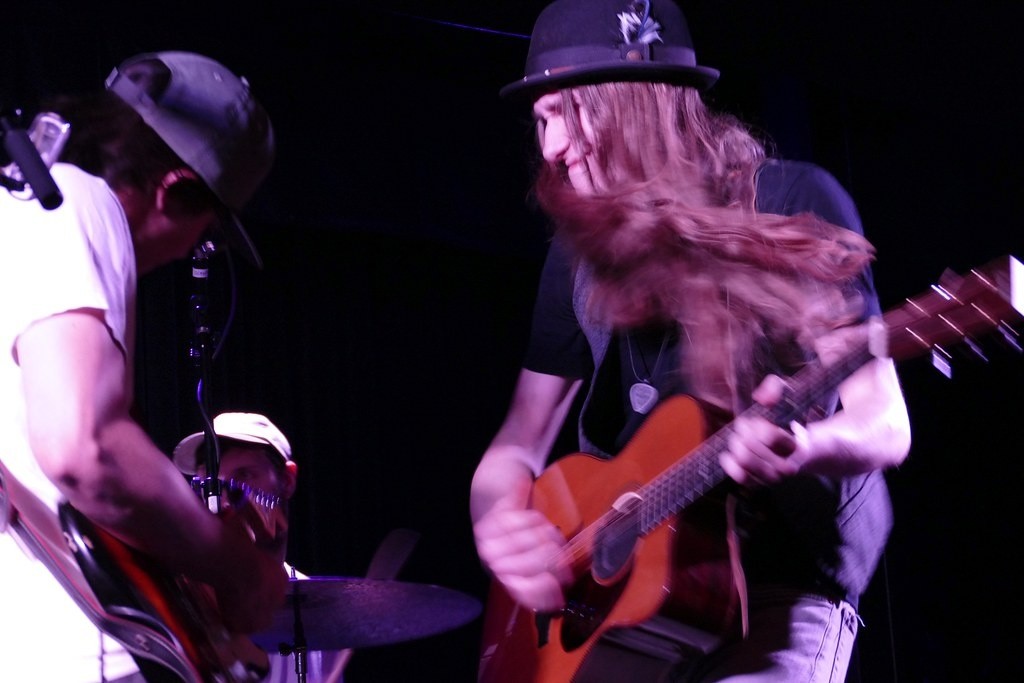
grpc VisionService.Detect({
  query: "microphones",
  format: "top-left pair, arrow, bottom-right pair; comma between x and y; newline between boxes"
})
0,97 -> 71,210
190,237 -> 217,376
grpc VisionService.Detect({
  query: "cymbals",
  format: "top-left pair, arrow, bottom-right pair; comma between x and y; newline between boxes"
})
252,574 -> 482,654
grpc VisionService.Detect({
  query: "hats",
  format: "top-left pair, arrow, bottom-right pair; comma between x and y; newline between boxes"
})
104,53 -> 273,267
173,413 -> 292,478
498,1 -> 722,102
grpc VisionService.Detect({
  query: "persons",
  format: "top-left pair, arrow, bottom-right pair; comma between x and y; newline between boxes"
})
145,409 -> 362,683
464,1 -> 914,683
0,49 -> 295,683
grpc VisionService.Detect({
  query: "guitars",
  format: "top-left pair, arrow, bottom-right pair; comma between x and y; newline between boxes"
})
475,253 -> 1022,683
